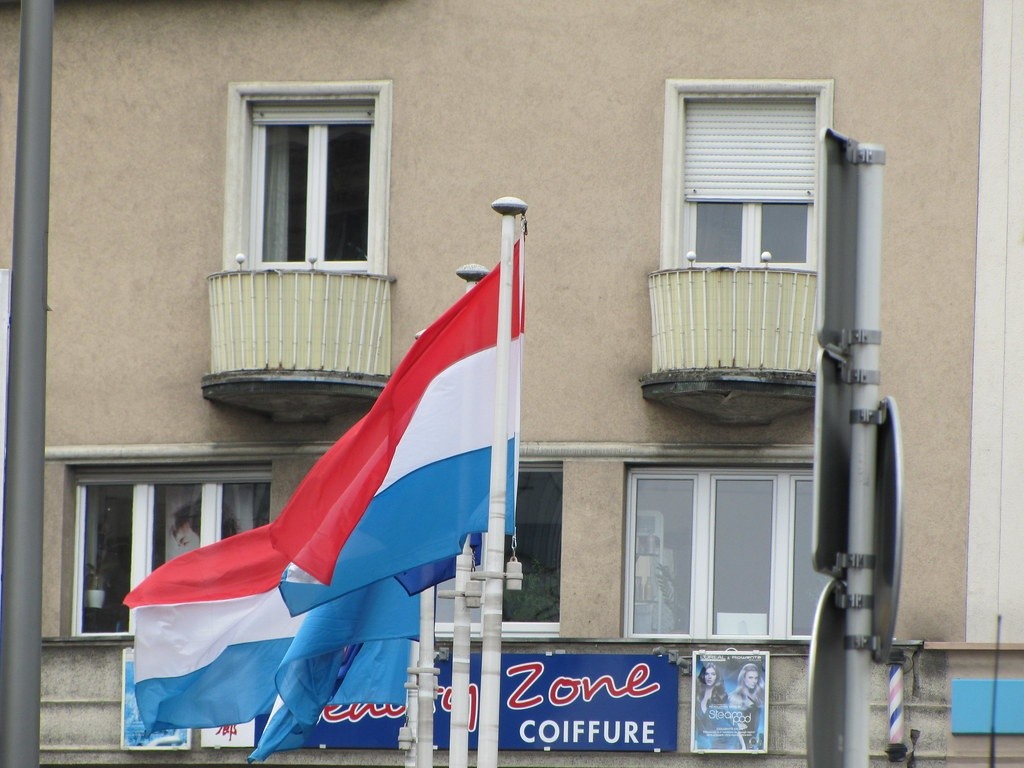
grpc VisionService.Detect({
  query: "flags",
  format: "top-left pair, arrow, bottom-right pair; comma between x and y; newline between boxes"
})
122,239 -> 526,764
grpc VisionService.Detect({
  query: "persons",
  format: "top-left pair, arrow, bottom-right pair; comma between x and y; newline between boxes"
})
168,496 -> 239,555
696,662 -> 728,736
729,663 -> 764,749
